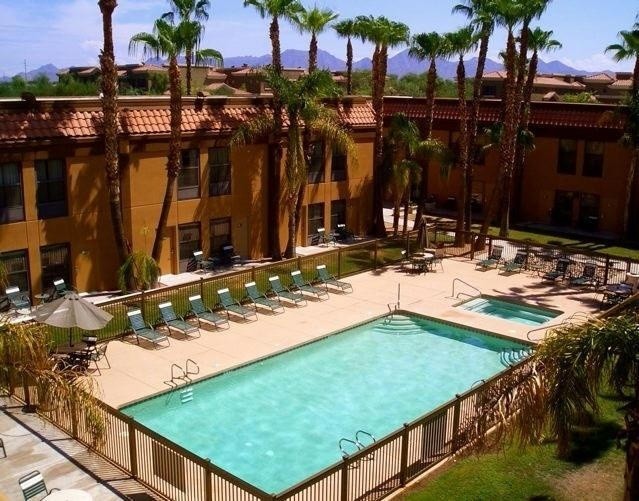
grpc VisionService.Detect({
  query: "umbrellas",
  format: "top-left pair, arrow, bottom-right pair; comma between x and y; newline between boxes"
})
28,292 -> 116,350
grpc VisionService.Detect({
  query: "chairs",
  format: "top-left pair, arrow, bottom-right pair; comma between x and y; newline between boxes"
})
19,470 -> 60,501
475,245 -> 639,308
400,248 -> 443,275
